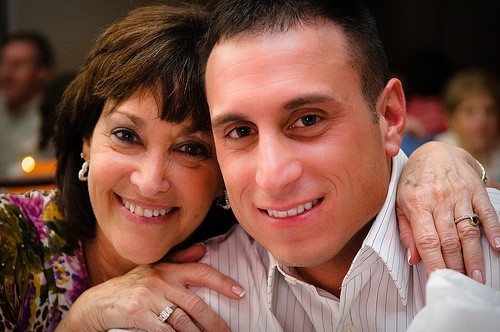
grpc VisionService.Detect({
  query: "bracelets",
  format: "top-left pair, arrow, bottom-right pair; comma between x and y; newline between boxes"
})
475,160 -> 488,185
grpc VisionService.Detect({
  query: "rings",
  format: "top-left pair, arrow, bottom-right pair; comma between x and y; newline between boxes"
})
158,304 -> 178,323
454,213 -> 480,226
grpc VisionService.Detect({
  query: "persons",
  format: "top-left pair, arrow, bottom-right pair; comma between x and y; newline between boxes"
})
1,3 -> 500,332
396,68 -> 500,186
108,0 -> 500,332
0,29 -> 60,162
